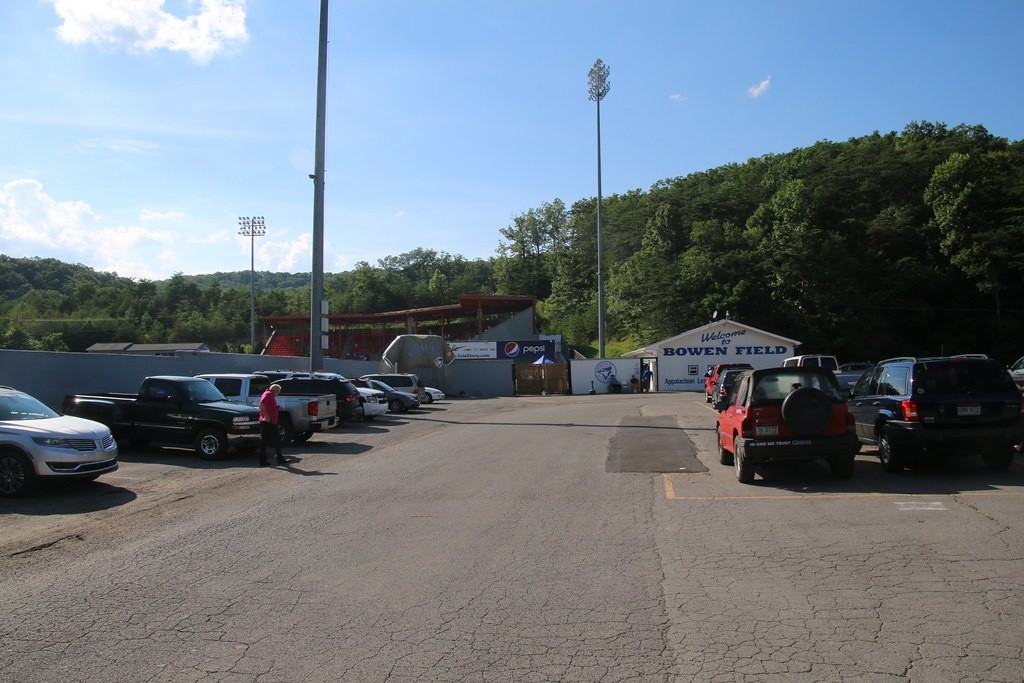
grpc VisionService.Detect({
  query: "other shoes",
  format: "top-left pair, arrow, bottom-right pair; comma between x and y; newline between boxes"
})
278,458 -> 291,464
643,391 -> 648,393
260,461 -> 271,466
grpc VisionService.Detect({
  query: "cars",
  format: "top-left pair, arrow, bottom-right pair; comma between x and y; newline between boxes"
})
253,371 -> 363,427
269,378 -> 352,428
1007,355 -> 1024,395
355,387 -> 389,423
347,379 -> 420,414
421,387 -> 446,404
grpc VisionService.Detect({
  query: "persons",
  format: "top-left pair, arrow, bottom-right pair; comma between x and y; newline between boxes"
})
631,375 -> 639,393
259,384 -> 291,467
608,375 -> 620,393
642,364 -> 653,392
708,367 -> 712,376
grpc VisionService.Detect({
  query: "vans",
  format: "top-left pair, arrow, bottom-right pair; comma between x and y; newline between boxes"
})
356,374 -> 425,408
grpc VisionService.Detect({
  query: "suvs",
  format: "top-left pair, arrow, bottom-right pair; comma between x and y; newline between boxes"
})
711,367 -> 754,409
716,366 -> 858,483
0,385 -> 118,498
703,363 -> 754,403
846,354 -> 1024,472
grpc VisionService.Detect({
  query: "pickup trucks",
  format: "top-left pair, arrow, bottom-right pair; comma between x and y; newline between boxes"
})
781,354 -> 862,391
62,376 -> 261,460
193,374 -> 340,447
